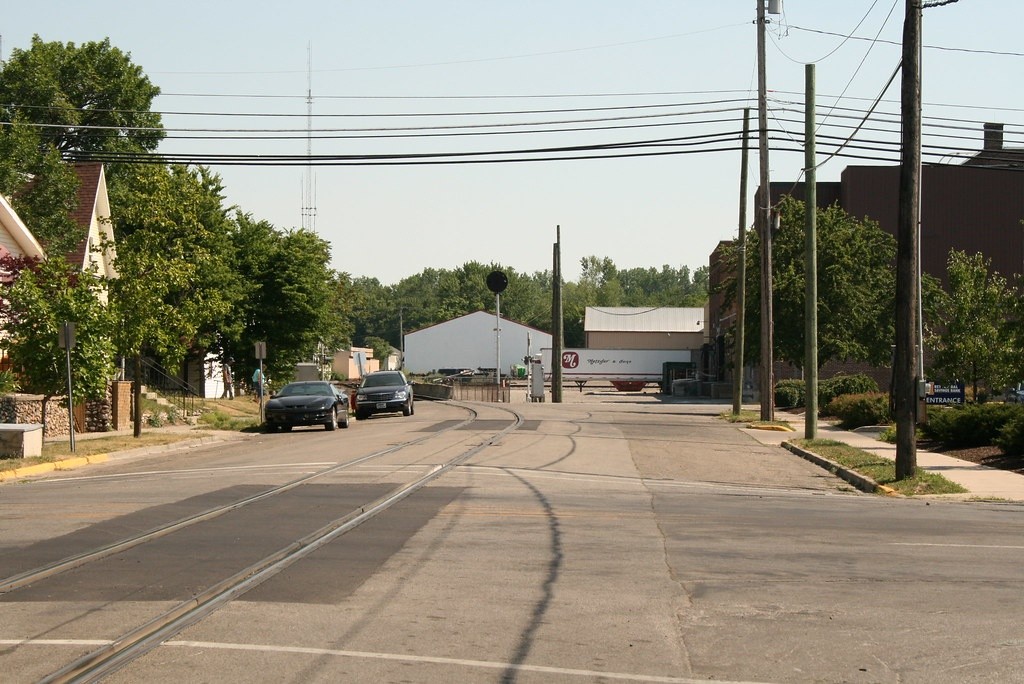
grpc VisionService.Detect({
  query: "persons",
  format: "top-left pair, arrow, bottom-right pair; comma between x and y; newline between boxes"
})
251,364 -> 267,404
219,357 -> 235,400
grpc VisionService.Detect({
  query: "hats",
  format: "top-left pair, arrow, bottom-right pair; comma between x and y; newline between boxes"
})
229,357 -> 235,362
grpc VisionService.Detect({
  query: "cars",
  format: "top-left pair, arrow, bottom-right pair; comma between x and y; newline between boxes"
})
265,381 -> 350,434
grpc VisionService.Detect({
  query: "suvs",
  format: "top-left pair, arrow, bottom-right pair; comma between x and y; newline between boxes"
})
354,370 -> 415,419
